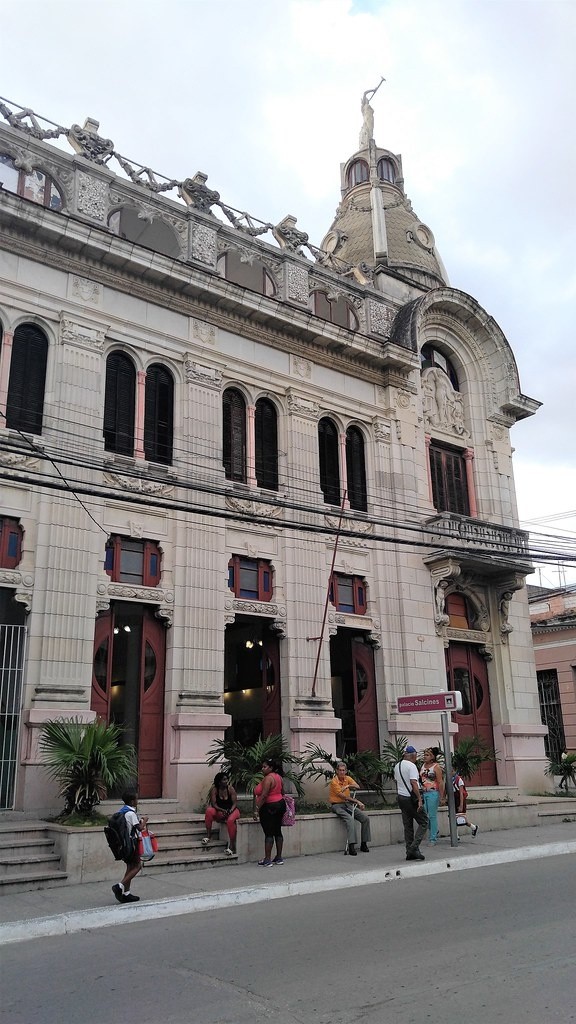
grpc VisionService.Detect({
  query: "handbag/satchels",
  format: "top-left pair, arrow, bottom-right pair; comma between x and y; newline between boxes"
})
410,789 -> 425,804
137,830 -> 158,862
281,796 -> 296,826
456,813 -> 468,826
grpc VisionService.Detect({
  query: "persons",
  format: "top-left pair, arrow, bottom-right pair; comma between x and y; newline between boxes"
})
112,790 -> 149,903
202,772 -> 240,856
330,762 -> 371,856
254,758 -> 286,867
394,746 -> 429,860
359,87 -> 377,149
420,747 -> 479,847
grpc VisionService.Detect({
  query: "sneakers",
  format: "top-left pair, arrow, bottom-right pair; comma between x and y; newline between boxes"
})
112,884 -> 140,903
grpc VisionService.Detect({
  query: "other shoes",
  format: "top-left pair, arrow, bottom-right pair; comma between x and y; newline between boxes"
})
226,846 -> 234,856
257,856 -> 283,867
406,848 -> 425,860
428,842 -> 438,848
202,838 -> 210,845
472,825 -> 479,838
558,783 -> 564,789
349,848 -> 357,856
360,844 -> 369,852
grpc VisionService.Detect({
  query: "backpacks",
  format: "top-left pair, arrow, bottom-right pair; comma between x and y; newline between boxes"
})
103,812 -> 135,861
457,776 -> 468,800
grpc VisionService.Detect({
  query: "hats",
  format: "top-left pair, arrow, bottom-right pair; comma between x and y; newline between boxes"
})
403,746 -> 417,753
